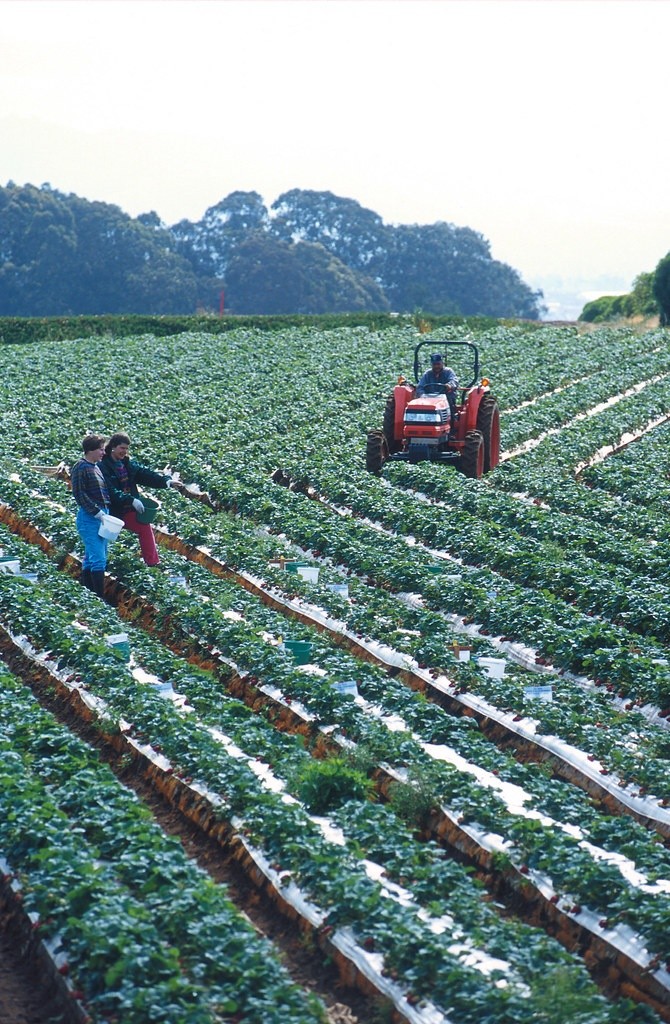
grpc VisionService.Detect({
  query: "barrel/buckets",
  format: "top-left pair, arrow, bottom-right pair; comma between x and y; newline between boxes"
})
284,559 -> 320,585
98,514 -> 125,540
478,658 -> 506,679
282,640 -> 312,665
106,633 -> 131,663
136,498 -> 159,524
327,585 -> 349,601
0,557 -> 37,586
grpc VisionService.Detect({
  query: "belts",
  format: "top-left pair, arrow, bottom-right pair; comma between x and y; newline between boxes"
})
101,504 -> 108,510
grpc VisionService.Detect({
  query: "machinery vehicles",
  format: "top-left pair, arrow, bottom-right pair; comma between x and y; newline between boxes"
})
364,339 -> 501,481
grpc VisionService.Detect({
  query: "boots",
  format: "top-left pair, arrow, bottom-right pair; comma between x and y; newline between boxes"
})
90,570 -> 119,608
82,570 -> 91,589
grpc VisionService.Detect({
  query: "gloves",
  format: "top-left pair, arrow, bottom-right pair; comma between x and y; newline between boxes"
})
131,498 -> 145,514
94,510 -> 106,526
165,480 -> 184,490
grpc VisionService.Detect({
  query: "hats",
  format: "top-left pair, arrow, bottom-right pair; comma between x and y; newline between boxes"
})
430,353 -> 442,362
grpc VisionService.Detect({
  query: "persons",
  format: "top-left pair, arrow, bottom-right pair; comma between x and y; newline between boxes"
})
69,432 -> 185,607
415,354 -> 459,441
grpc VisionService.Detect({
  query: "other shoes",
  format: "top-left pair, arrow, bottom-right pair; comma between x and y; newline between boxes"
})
451,435 -> 457,440
